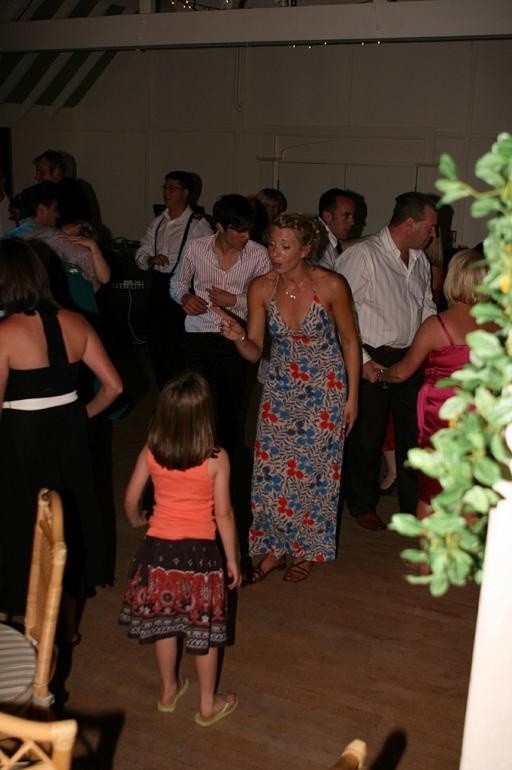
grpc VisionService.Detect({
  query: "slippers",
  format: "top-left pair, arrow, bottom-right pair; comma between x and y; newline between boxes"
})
195,693 -> 239,727
158,676 -> 189,713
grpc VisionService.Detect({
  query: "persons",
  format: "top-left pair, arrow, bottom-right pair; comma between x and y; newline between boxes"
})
331,738 -> 367,770
376,251 -> 491,580
333,192 -> 444,530
122,376 -> 240,726
2,146 -> 122,702
135,172 -> 361,496
212,213 -> 360,580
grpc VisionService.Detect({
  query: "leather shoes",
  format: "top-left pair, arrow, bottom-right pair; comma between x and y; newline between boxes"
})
358,512 -> 386,532
336,736 -> 369,768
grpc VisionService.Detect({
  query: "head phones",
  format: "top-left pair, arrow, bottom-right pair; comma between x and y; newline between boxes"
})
114,235 -> 141,247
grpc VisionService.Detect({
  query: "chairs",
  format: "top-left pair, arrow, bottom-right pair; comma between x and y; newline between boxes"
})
0,714 -> 78,770
0,478 -> 71,760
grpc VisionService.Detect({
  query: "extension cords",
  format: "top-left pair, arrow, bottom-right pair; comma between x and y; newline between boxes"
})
112,279 -> 144,290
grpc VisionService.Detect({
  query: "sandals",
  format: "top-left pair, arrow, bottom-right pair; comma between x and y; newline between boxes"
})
250,561 -> 287,585
284,561 -> 313,584
